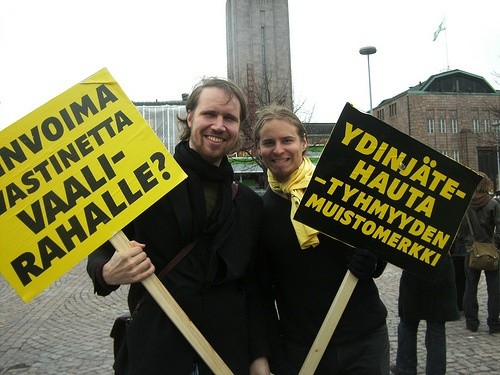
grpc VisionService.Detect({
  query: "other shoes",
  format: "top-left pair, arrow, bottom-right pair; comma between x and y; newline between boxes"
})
390,362 -> 401,375
487,326 -> 499,335
466,322 -> 479,331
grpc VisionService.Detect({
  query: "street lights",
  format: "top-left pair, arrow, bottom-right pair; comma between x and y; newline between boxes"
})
359,46 -> 377,115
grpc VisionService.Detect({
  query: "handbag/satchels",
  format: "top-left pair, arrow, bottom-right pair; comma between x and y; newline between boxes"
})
109,316 -> 132,375
468,241 -> 499,270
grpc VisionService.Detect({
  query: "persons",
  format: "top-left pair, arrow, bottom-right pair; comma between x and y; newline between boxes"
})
248,106 -> 389,375
87,78 -> 500,375
